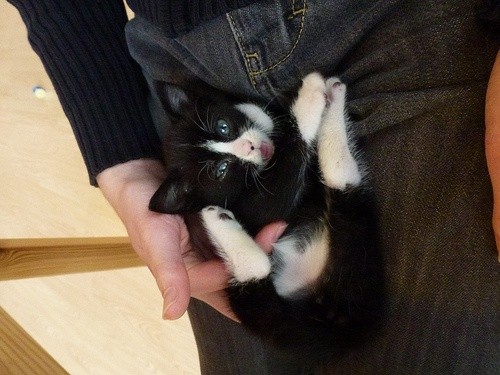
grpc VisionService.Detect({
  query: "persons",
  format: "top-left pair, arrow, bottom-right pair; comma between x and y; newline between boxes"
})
4,1 -> 500,375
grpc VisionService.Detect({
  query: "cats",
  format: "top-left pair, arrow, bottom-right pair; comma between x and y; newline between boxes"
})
147,70 -> 388,351
